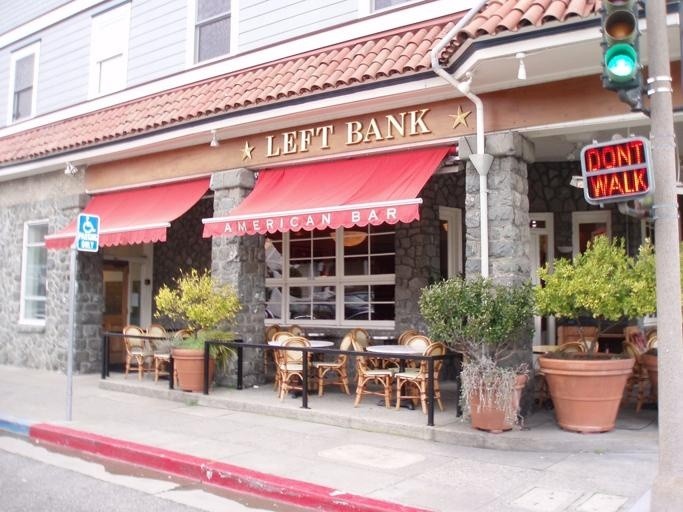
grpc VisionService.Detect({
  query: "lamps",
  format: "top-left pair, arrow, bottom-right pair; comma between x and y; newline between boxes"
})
456,75 -> 472,94
515,53 -> 526,81
209,130 -> 219,148
64,161 -> 78,176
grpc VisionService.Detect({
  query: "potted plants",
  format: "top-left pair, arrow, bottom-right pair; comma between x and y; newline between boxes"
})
154,268 -> 243,392
539,233 -> 657,433
418,273 -> 537,432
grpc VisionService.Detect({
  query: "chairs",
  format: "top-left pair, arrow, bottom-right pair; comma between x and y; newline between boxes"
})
266,325 -> 446,414
539,325 -> 657,413
123,324 -> 192,382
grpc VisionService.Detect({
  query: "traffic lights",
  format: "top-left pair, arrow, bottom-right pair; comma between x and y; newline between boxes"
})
602,0 -> 636,85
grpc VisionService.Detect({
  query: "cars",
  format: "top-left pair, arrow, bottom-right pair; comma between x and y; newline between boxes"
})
290,290 -> 374,320
